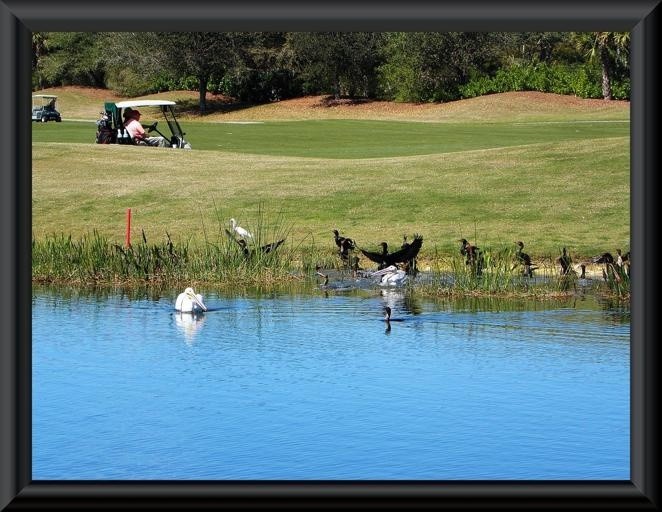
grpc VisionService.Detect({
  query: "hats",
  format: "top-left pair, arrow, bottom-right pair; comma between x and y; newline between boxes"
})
123,107 -> 142,117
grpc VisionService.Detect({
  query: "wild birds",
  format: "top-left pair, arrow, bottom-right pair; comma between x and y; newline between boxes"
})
382,306 -> 405,322
174,287 -> 207,313
331,229 -> 424,284
225,229 -> 284,262
559,248 -> 630,291
514,241 -> 540,279
457,239 -> 484,275
230,218 -> 252,239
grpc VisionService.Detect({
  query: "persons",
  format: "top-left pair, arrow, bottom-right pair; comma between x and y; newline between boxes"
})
120,106 -> 167,147
